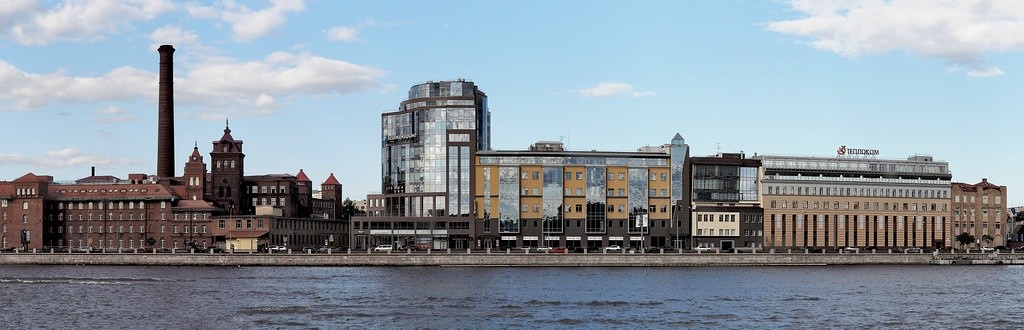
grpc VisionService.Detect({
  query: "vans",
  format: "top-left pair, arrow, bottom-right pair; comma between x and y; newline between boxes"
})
375,245 -> 392,253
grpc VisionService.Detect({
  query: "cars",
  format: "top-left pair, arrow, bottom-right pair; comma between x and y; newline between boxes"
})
206,246 -> 225,253
401,246 -> 419,252
302,247 -> 320,254
994,246 -> 1006,252
965,247 -> 978,250
695,245 -> 711,252
939,246 -> 959,251
264,245 -> 287,253
320,247 -> 339,253
77,246 -> 93,252
980,246 -> 994,251
661,246 -> 673,253
13,247 -> 26,251
904,247 -> 920,252
606,245 -> 620,251
188,245 -> 204,253
845,247 -> 860,251
537,246 -> 552,252
550,247 -> 565,253
